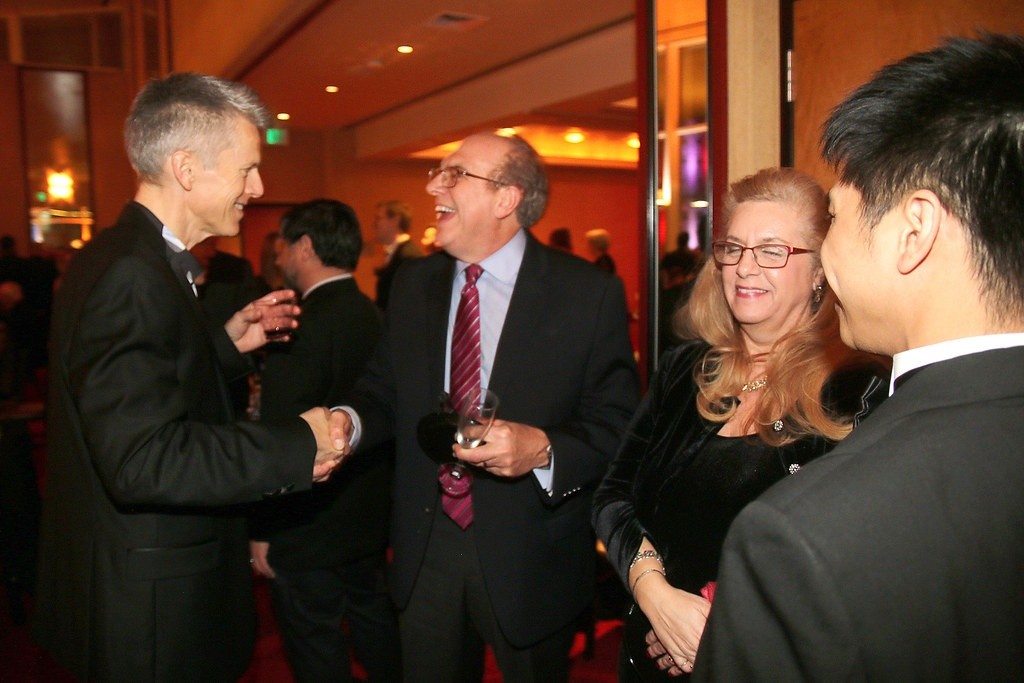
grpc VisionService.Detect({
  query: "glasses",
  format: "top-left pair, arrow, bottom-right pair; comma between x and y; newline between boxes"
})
713,240 -> 817,268
430,168 -> 508,190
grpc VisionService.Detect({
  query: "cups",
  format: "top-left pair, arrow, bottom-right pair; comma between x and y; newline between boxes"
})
253,297 -> 295,338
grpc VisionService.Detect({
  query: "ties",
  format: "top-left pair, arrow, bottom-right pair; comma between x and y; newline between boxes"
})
441,263 -> 485,530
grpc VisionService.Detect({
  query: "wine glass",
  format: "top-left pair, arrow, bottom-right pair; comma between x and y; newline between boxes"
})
437,387 -> 498,491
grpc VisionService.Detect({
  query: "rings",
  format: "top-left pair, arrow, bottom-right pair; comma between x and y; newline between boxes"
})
483,461 -> 487,467
679,660 -> 689,668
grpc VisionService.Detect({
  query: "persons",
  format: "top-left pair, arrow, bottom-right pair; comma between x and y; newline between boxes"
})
249,199 -> 402,683
688,33 -> 1024,683
371,198 -> 421,262
30,74 -> 350,683
328,134 -> 642,683
422,223 -> 442,252
588,228 -> 617,273
552,227 -> 572,249
591,166 -> 890,683
662,231 -> 696,270
191,232 -> 303,319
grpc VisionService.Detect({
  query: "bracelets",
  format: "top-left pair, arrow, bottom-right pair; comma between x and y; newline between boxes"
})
542,445 -> 552,470
631,550 -> 666,575
633,569 -> 664,604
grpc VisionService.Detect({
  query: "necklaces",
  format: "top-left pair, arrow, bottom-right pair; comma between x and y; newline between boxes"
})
742,379 -> 766,392
